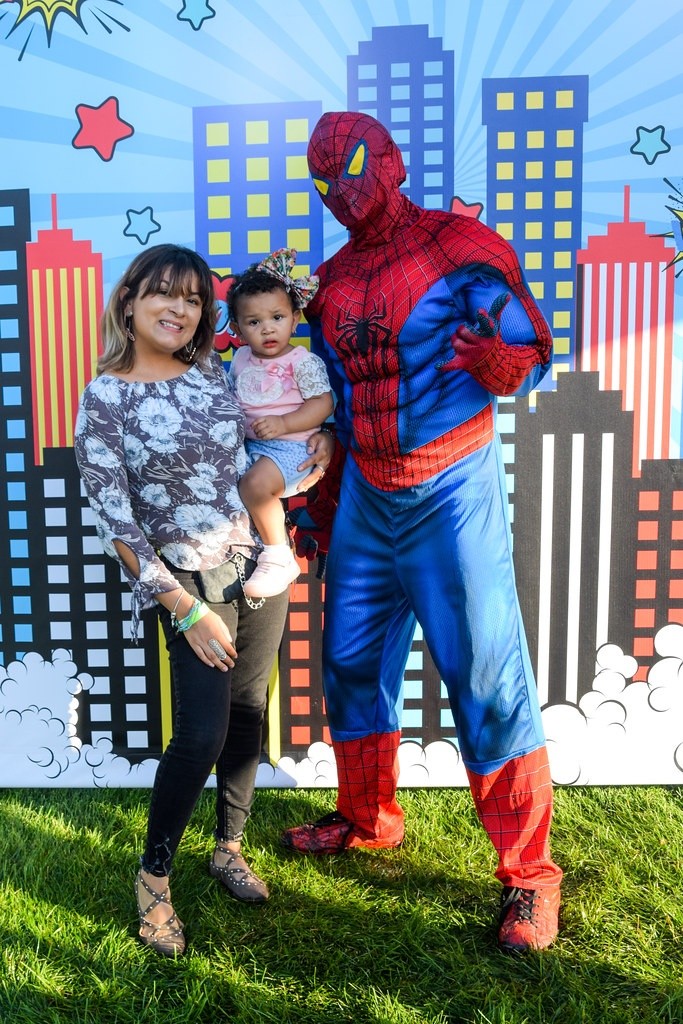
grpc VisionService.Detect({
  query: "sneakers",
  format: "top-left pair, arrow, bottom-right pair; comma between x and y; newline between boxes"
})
497,886 -> 560,953
281,811 -> 404,856
243,549 -> 301,597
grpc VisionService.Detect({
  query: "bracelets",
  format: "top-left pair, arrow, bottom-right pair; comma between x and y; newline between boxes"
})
322,428 -> 335,439
171,588 -> 184,627
176,598 -> 208,633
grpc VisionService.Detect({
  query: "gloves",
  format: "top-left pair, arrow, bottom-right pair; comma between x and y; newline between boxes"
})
435,291 -> 513,371
286,501 -> 332,580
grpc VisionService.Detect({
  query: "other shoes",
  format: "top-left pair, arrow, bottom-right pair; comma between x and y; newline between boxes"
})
210,841 -> 271,904
135,868 -> 185,959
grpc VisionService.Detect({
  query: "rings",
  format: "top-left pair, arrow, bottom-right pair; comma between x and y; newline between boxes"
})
208,638 -> 226,660
316,466 -> 325,478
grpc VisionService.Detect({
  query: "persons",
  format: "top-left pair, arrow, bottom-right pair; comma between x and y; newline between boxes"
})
226,269 -> 334,598
75,244 -> 291,953
286,111 -> 562,947
